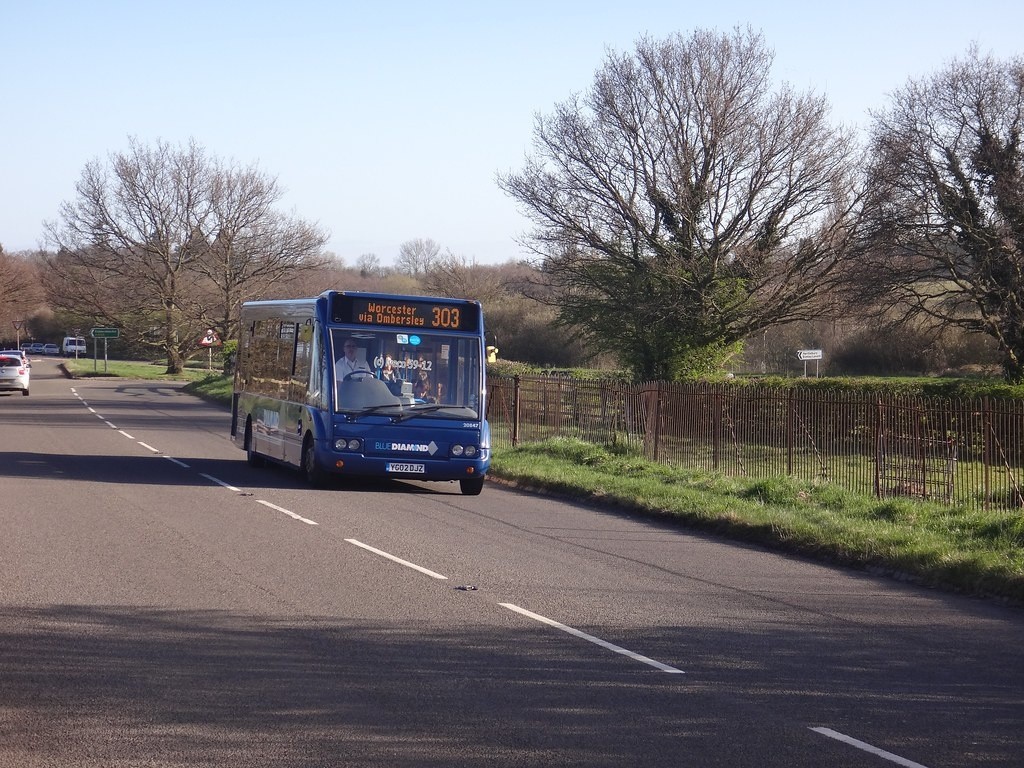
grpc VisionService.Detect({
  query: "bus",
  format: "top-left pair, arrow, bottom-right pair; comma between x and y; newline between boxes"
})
231,289 -> 499,496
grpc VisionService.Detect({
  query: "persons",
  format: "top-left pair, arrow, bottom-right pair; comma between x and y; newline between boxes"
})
335,339 -> 374,382
379,355 -> 440,405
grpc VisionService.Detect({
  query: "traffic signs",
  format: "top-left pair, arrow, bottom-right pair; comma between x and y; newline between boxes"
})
88,327 -> 119,338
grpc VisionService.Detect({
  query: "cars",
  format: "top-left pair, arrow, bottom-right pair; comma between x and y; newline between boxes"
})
42,343 -> 61,357
29,343 -> 45,355
0,348 -> 33,396
20,343 -> 32,354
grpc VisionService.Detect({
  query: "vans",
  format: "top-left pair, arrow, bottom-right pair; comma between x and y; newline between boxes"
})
62,336 -> 88,358
